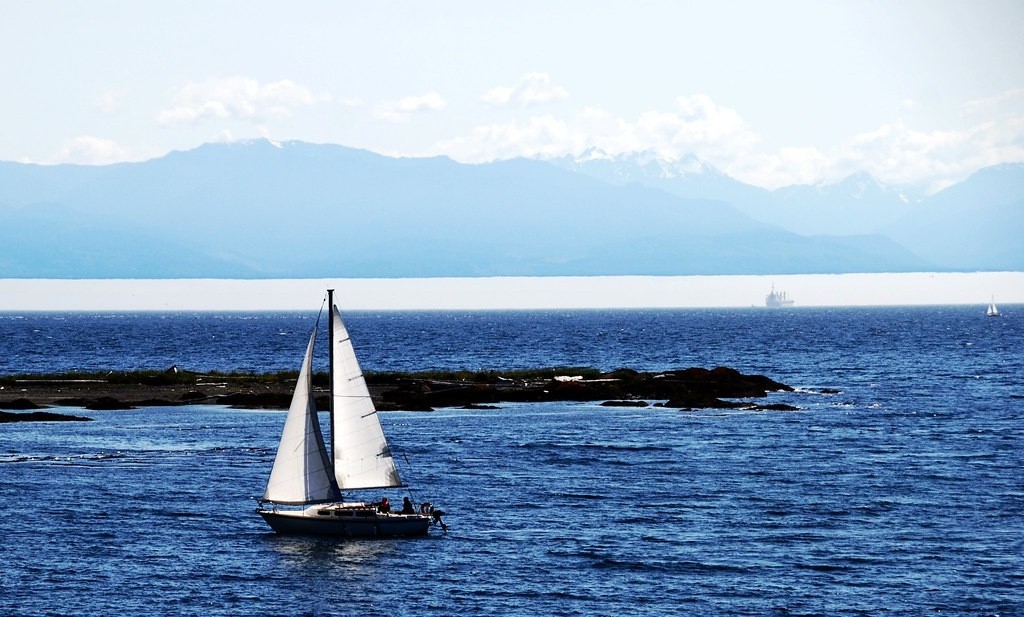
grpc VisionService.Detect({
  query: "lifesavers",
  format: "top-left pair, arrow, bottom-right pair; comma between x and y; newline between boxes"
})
421,501 -> 434,515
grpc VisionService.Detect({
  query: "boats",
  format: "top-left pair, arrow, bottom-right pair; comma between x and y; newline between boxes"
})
766,284 -> 794,308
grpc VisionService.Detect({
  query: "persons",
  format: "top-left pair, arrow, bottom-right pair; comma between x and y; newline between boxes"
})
379,497 -> 390,514
394,497 -> 414,514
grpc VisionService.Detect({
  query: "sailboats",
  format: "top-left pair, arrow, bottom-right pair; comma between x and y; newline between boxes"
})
984,295 -> 999,317
252,290 -> 451,539
